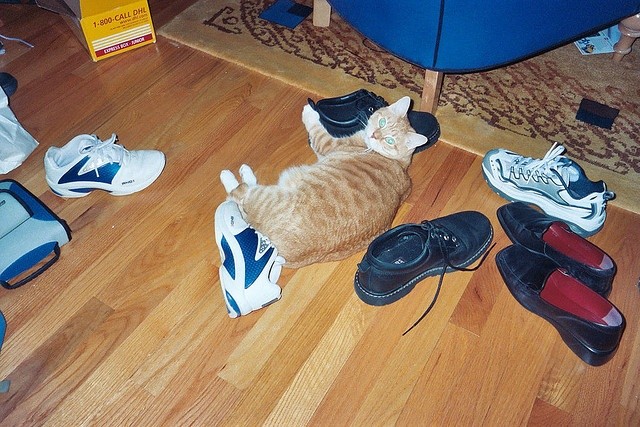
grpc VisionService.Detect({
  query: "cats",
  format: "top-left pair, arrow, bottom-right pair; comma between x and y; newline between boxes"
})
219,95 -> 429,270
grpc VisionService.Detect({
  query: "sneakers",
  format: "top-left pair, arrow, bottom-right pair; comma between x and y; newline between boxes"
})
214,200 -> 286,318
42,133 -> 166,198
481,142 -> 616,238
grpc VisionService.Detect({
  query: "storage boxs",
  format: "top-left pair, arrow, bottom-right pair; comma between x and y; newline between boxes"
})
35,0 -> 156,63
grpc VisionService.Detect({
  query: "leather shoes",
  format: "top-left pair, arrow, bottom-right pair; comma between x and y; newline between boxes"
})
354,210 -> 496,336
496,201 -> 617,298
495,242 -> 626,367
304,88 -> 440,154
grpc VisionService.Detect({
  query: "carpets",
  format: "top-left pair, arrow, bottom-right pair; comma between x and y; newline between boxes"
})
155,0 -> 639,213
